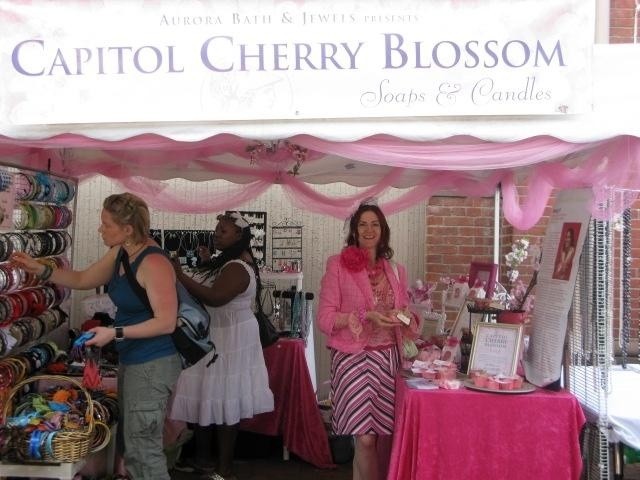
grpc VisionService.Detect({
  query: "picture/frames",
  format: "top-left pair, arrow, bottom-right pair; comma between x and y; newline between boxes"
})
449,298 -> 489,374
467,322 -> 523,380
469,262 -> 496,303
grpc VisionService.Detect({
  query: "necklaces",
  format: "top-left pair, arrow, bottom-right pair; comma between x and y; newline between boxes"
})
128,237 -> 147,259
367,258 -> 383,278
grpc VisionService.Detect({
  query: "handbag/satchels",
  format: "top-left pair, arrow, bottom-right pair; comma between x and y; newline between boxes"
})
402,334 -> 418,360
170,274 -> 214,369
253,311 -> 279,349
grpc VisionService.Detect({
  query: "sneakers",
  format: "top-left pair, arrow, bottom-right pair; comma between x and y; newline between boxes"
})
172,459 -> 214,473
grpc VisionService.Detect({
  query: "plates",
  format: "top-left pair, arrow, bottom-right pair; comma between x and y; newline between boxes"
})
466,377 -> 538,395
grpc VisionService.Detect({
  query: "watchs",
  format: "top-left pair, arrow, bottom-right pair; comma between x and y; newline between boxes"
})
113,326 -> 125,342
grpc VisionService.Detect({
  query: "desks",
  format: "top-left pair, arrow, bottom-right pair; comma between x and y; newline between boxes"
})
387,354 -> 585,480
241,338 -> 340,469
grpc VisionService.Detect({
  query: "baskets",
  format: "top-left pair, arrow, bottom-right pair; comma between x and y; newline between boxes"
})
2,374 -> 96,464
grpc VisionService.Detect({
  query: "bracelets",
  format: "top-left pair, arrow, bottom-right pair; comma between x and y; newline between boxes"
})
37,264 -> 52,283
359,305 -> 369,324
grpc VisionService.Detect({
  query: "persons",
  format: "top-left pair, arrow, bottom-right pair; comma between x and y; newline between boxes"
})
8,192 -> 185,480
163,210 -> 275,480
552,228 -> 576,280
316,203 -> 424,480
